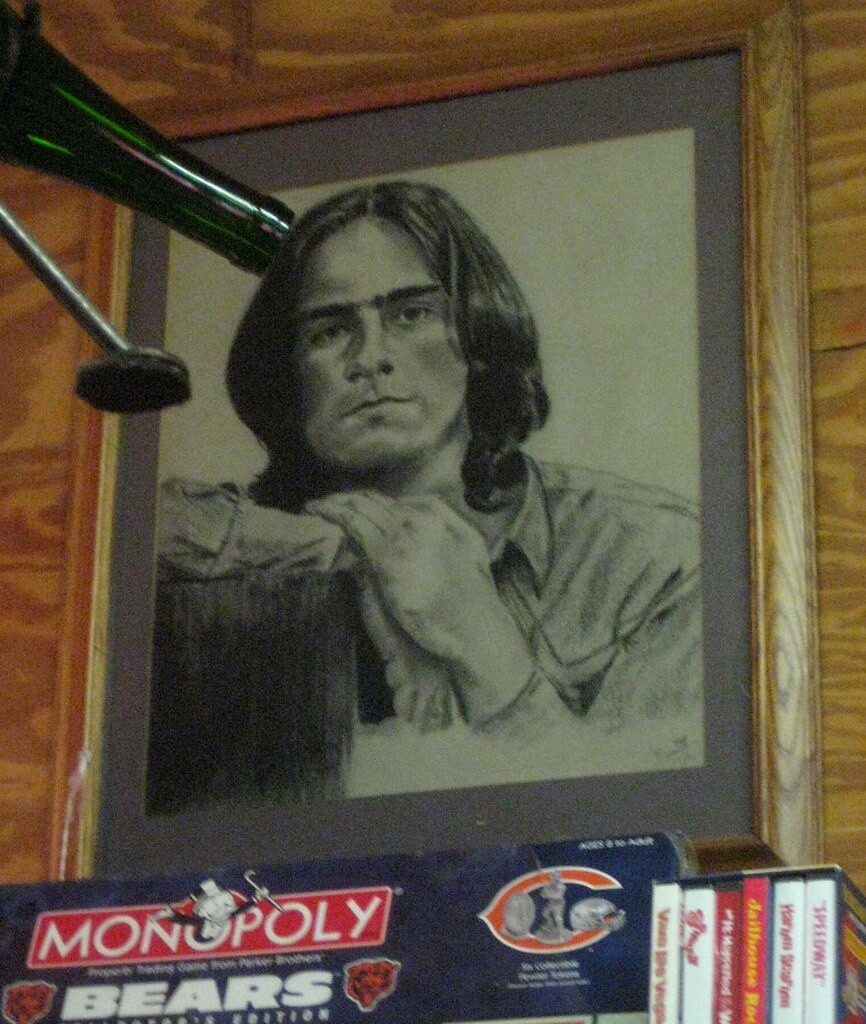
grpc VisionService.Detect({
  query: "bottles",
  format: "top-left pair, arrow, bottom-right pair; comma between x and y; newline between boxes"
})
0,0 -> 297,275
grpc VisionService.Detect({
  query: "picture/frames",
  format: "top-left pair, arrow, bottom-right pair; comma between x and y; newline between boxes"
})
44,1 -> 827,891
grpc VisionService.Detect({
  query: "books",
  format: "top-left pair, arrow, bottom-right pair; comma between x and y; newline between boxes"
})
647,862 -> 866,1023
